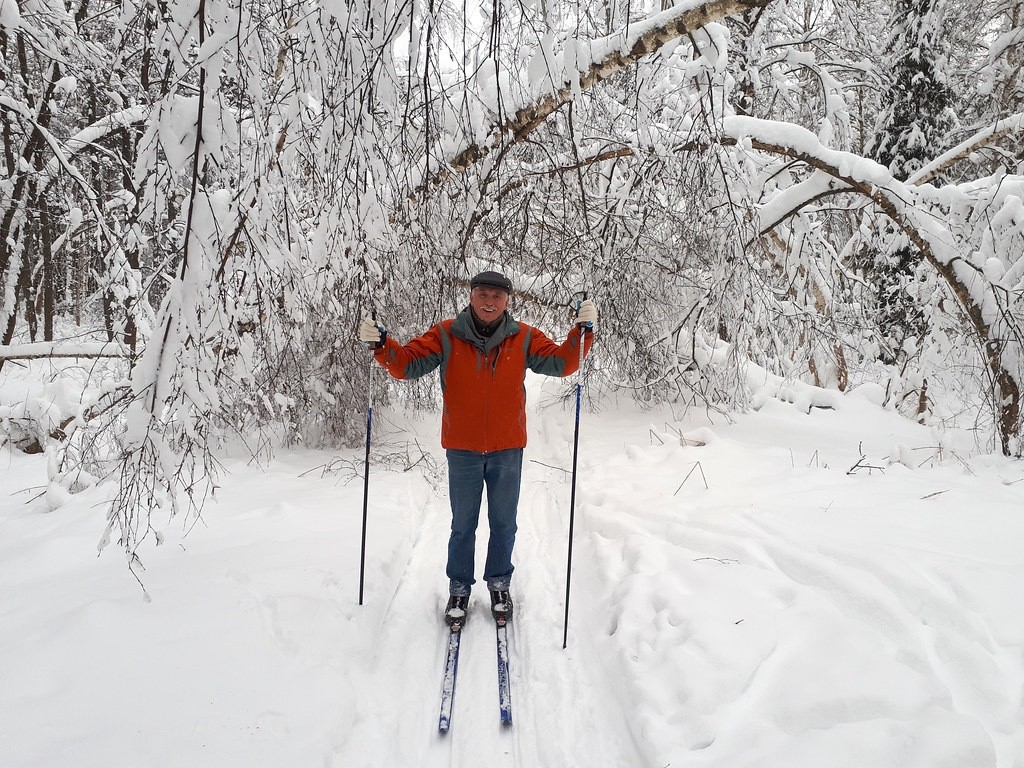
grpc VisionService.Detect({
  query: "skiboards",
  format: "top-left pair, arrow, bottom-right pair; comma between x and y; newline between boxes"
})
436,624 -> 513,735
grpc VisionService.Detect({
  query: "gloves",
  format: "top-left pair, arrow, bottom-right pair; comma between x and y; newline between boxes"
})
573,299 -> 597,326
360,318 -> 386,342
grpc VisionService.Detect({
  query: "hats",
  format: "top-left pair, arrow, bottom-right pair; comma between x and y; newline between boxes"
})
470,271 -> 513,295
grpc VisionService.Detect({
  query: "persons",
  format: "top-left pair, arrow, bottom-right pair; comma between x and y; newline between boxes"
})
359,271 -> 598,627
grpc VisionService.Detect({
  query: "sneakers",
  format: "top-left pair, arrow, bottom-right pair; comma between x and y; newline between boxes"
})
444,578 -> 472,626
487,577 -> 514,623
